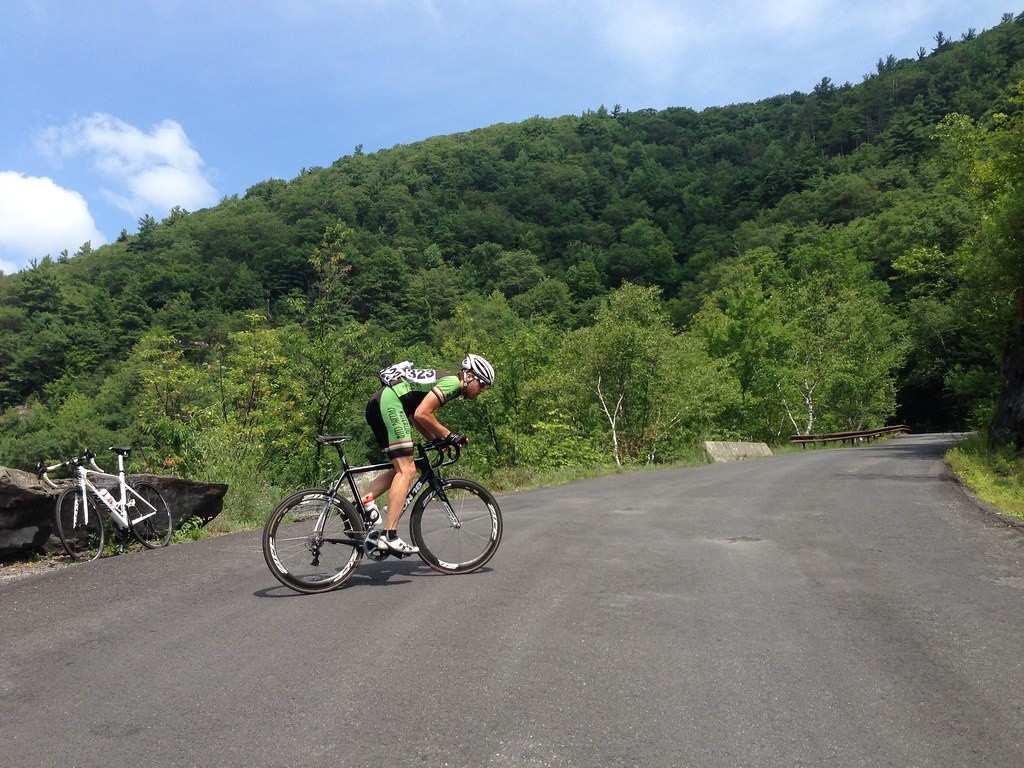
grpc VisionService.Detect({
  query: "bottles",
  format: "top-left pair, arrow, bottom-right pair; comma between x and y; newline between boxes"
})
98,489 -> 116,507
363,496 -> 383,525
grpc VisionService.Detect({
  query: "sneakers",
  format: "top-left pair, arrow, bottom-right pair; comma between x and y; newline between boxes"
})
378,535 -> 420,555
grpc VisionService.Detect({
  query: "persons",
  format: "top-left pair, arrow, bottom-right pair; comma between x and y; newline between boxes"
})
359,353 -> 495,555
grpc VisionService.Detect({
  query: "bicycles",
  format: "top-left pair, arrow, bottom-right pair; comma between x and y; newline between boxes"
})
35,447 -> 173,564
263,434 -> 503,594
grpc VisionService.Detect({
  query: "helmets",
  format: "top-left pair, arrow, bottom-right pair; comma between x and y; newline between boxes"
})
462,353 -> 494,386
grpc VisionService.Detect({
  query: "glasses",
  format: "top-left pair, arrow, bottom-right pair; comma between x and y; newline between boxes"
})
473,374 -> 486,388
